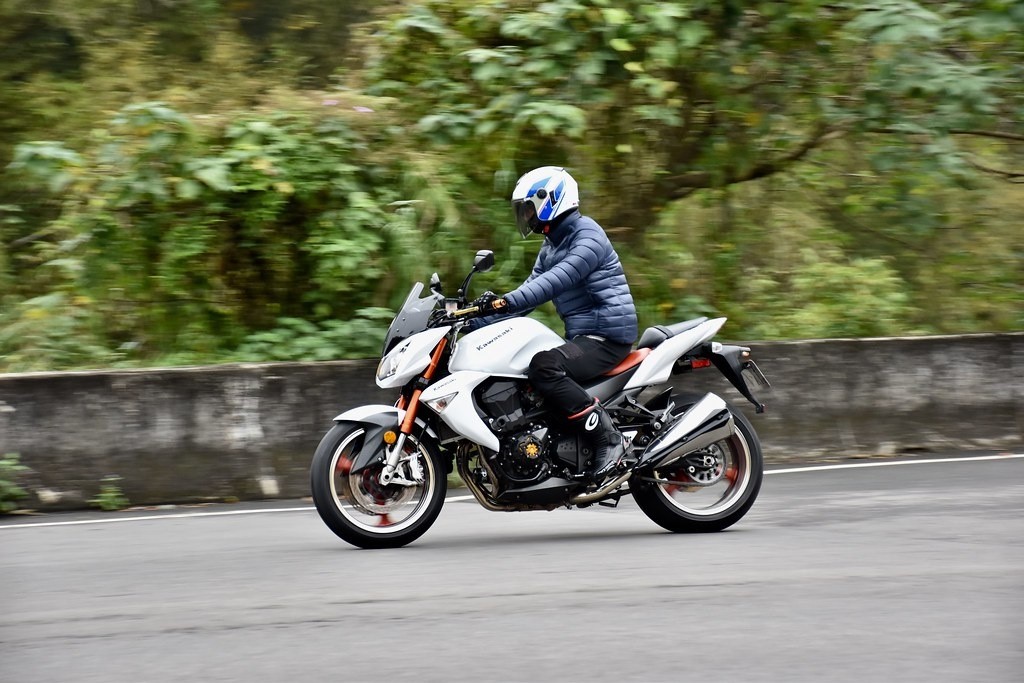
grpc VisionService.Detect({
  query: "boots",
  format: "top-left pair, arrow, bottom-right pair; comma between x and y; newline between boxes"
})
568,397 -> 632,504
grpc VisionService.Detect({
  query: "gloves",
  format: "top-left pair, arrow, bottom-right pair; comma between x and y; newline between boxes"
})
473,291 -> 498,314
433,308 -> 448,322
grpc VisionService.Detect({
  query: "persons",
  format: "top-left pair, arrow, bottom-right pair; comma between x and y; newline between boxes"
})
452,165 -> 638,507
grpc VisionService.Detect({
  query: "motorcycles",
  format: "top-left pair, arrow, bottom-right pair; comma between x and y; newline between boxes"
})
311,249 -> 771,549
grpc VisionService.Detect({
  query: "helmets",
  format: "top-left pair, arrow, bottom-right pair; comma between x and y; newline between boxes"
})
511,166 -> 579,221
